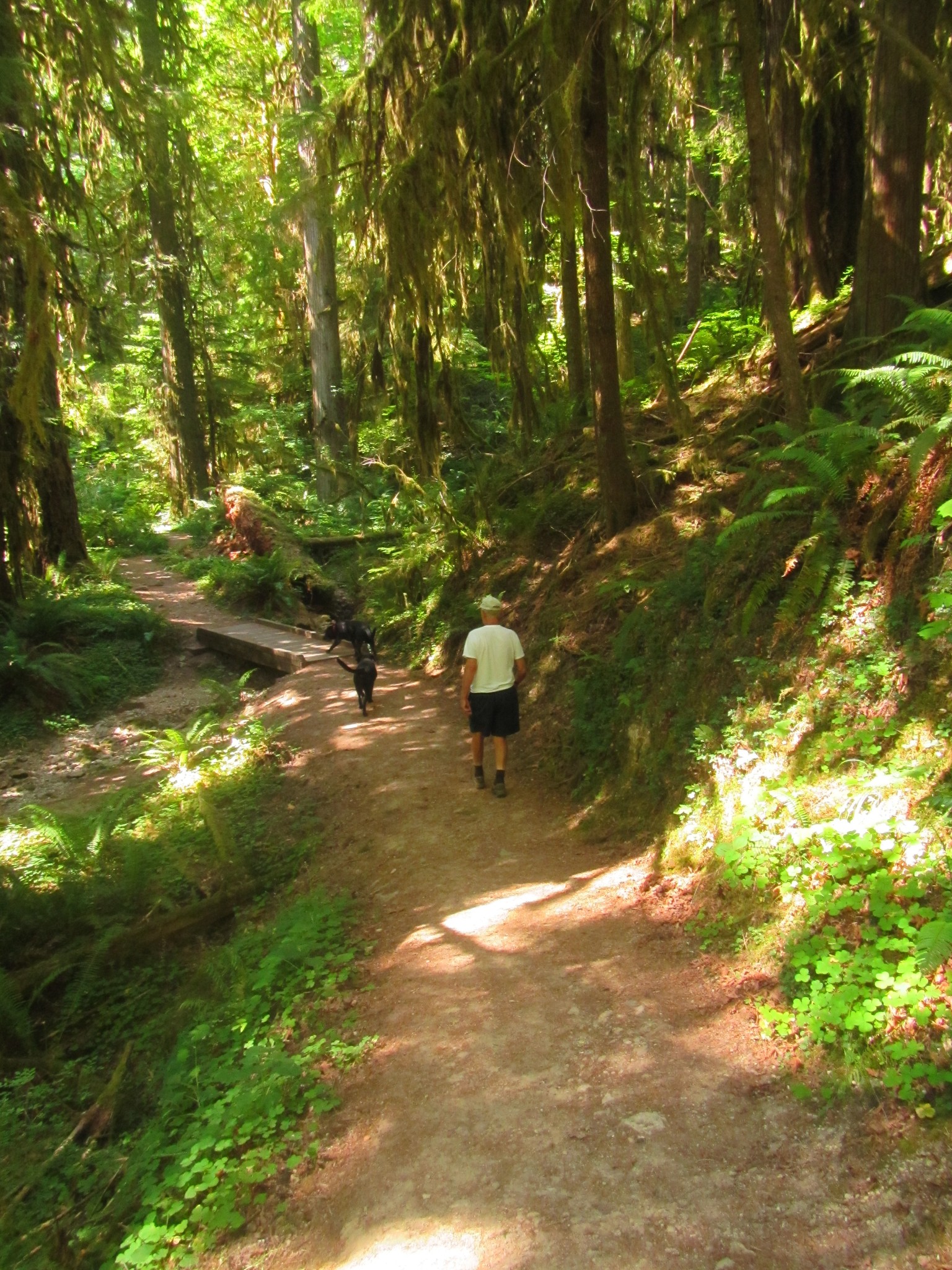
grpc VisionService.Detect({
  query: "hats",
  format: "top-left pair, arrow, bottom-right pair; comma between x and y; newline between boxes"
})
475,596 -> 502,610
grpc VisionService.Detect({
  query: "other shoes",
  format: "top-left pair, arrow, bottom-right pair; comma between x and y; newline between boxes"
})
490,782 -> 507,798
472,770 -> 485,789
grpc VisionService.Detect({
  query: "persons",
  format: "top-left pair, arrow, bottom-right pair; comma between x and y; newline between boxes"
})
460,594 -> 527,799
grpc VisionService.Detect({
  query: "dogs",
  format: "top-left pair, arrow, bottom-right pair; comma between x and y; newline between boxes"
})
336,652 -> 378,718
323,620 -> 376,662
